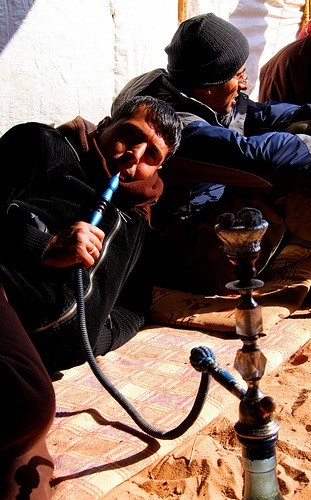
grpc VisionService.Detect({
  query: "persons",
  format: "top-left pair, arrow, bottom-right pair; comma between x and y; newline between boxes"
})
0,97 -> 182,377
112,12 -> 311,292
0,284 -> 59,499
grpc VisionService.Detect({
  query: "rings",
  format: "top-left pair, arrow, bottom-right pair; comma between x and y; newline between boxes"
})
89,246 -> 97,255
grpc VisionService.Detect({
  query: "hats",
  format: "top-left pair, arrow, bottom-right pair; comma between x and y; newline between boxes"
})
165,13 -> 249,89
